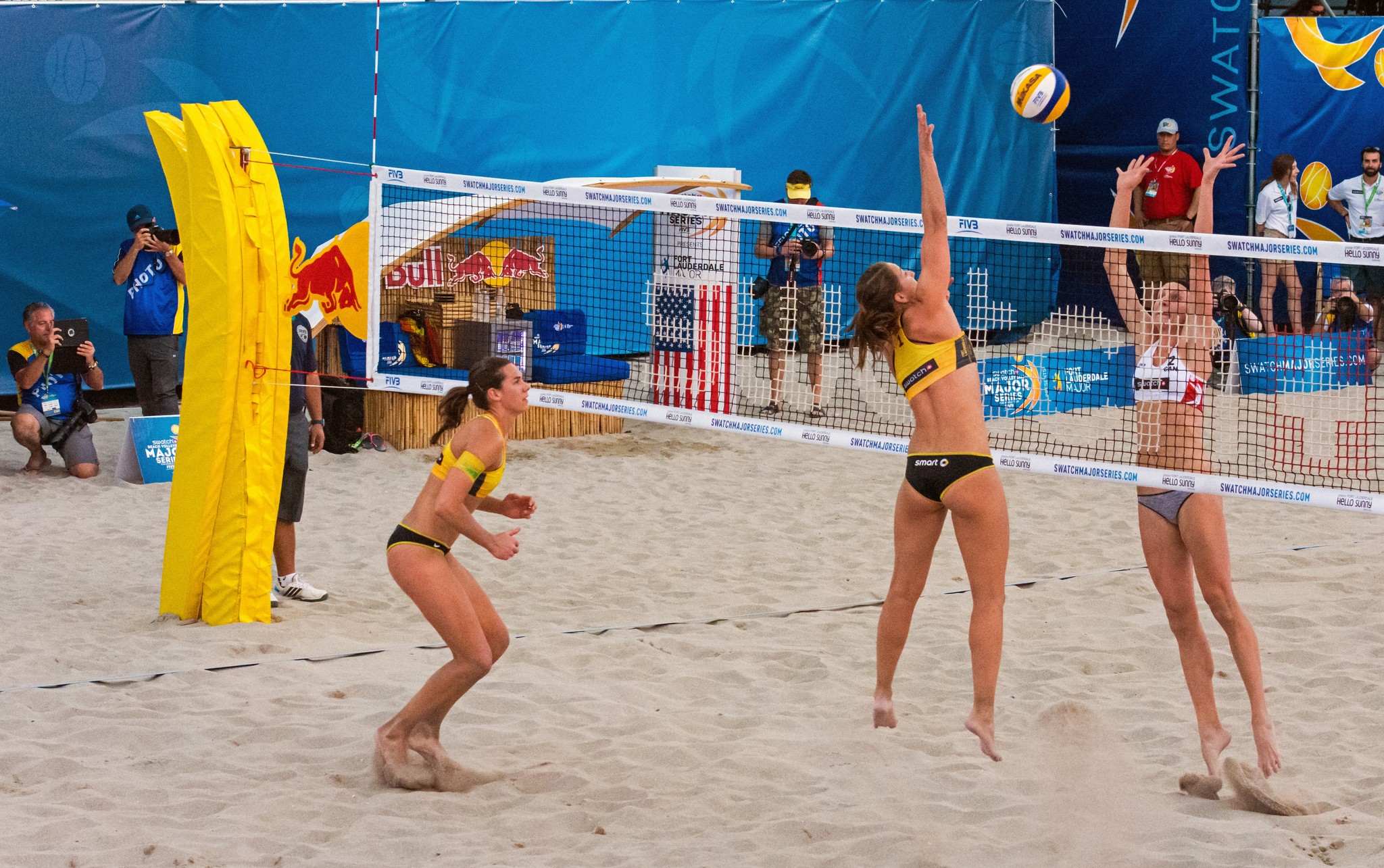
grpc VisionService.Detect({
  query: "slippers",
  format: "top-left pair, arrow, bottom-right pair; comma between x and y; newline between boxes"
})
759,401 -> 781,416
360,432 -> 388,452
810,402 -> 826,418
19,457 -> 52,477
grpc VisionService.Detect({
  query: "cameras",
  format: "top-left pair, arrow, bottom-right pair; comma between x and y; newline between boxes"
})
1212,289 -> 1238,310
47,398 -> 98,453
795,237 -> 817,257
142,226 -> 180,250
1330,296 -> 1358,315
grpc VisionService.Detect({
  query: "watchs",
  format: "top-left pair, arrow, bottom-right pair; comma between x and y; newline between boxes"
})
311,419 -> 324,427
776,247 -> 780,256
163,250 -> 173,257
1181,213 -> 1193,222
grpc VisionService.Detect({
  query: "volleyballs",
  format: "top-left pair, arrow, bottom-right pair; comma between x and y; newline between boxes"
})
1009,63 -> 1071,124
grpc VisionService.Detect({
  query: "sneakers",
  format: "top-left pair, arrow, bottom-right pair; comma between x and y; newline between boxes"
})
273,573 -> 328,602
270,591 -> 278,607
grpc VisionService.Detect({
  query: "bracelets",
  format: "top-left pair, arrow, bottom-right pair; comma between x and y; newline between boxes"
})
37,350 -> 50,358
1342,213 -> 1349,218
86,361 -> 98,370
818,250 -> 825,260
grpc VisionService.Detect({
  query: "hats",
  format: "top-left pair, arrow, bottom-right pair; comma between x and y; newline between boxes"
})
126,203 -> 154,231
1156,118 -> 1178,135
785,182 -> 811,199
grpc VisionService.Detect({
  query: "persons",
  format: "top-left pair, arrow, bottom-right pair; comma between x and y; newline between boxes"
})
1102,134 -> 1283,788
754,170 -> 835,418
843,105 -> 1011,761
1255,154 -> 1304,337
270,313 -> 328,608
1326,146 -> 1384,342
374,359 -> 537,776
1133,118 -> 1203,315
7,302 -> 103,478
1209,275 -> 1380,373
113,204 -> 188,416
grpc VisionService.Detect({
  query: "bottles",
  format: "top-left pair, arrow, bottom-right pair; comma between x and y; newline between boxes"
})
479,291 -> 490,322
496,289 -> 506,320
472,288 -> 481,318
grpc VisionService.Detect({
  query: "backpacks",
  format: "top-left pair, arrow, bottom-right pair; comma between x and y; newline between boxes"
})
316,373 -> 365,455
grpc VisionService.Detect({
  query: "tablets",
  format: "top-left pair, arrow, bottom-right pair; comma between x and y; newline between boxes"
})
50,318 -> 89,375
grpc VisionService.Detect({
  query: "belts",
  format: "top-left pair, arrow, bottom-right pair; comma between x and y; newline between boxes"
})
1145,215 -> 1185,225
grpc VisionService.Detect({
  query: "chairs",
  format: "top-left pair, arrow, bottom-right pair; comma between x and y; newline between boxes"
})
336,320 -> 470,389
522,308 -> 631,384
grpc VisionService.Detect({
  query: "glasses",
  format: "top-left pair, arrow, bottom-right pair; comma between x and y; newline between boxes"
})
1364,146 -> 1381,152
